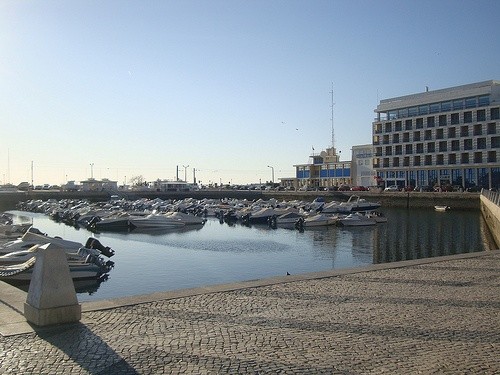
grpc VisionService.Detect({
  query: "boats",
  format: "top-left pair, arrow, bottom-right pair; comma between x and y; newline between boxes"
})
0,193 -> 390,264
433,205 -> 451,211
0,236 -> 116,281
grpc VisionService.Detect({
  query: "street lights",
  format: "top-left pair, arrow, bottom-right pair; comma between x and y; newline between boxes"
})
89,163 -> 94,181
267,166 -> 274,188
183,164 -> 190,191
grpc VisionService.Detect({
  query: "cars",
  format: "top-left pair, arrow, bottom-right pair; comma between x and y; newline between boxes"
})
232,183 -> 481,193
489,186 -> 500,192
